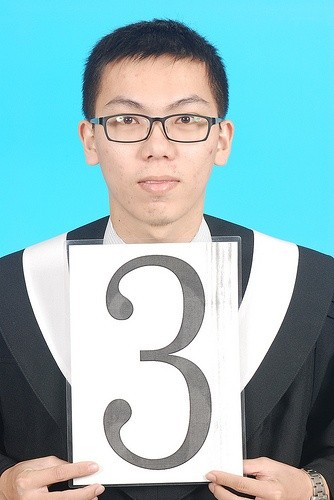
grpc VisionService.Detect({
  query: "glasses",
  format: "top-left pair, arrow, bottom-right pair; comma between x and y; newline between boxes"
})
88,113 -> 224,143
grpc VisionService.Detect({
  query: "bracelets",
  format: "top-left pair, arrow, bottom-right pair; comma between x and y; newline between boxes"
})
301,467 -> 329,500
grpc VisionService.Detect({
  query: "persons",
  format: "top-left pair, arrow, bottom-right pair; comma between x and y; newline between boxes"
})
0,18 -> 334,500
